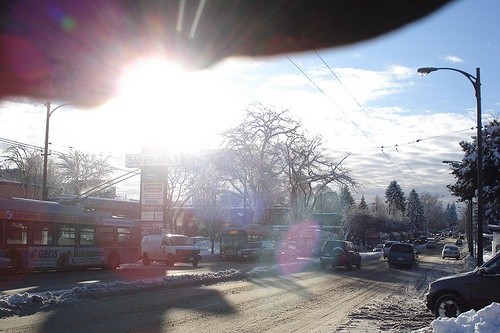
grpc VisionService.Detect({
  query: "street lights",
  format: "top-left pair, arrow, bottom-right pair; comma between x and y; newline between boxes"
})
241,169 -> 269,249
42,101 -> 86,200
417,66 -> 484,268
363,202 -> 377,253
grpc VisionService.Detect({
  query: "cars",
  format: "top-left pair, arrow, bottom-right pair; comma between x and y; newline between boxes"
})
387,243 -> 422,266
441,244 -> 460,260
373,231 -> 466,254
425,250 -> 500,320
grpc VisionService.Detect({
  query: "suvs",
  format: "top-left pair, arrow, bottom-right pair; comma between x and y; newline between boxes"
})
236,241 -> 275,263
141,234 -> 202,267
319,239 -> 362,271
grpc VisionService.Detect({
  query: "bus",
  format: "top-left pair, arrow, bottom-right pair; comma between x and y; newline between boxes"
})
0,167 -> 142,273
